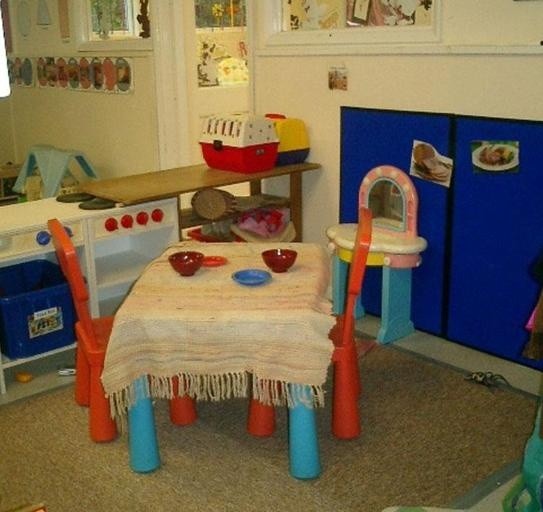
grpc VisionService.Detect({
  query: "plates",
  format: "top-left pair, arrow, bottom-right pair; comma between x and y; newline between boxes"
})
203,255 -> 227,267
232,268 -> 271,286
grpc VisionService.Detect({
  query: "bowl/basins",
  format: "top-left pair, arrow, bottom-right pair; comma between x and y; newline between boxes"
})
261,249 -> 298,273
168,250 -> 204,278
